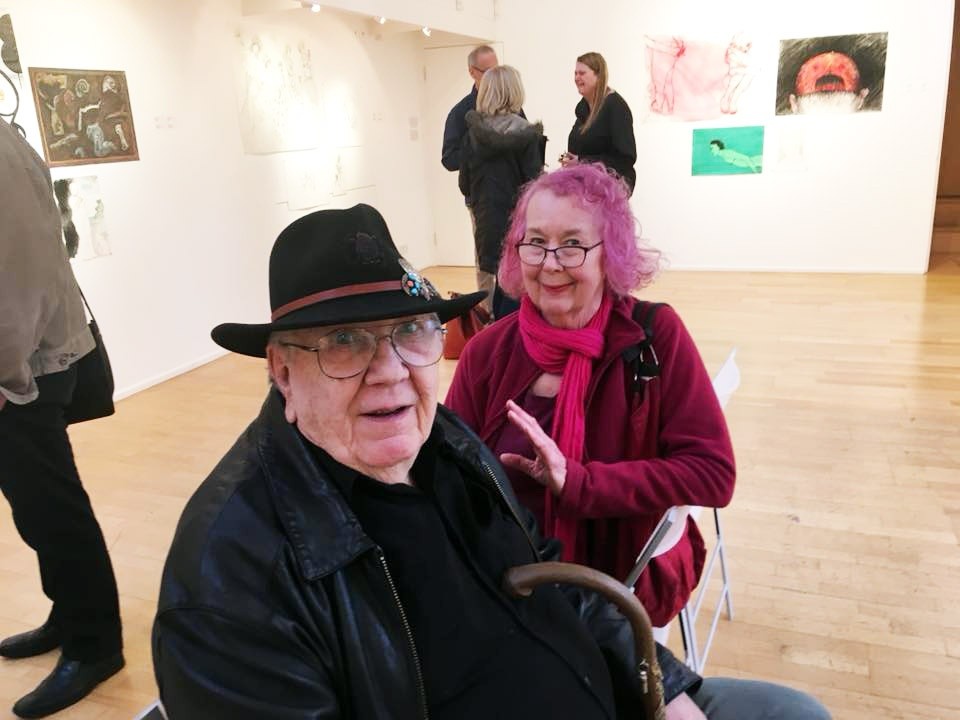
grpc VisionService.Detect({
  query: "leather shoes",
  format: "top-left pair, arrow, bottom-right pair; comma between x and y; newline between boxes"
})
1,604 -> 64,660
11,652 -> 127,719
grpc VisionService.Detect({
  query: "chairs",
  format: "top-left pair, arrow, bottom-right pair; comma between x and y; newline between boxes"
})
626,348 -> 742,676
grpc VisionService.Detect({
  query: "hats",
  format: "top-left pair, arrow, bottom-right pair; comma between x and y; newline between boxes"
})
211,202 -> 489,356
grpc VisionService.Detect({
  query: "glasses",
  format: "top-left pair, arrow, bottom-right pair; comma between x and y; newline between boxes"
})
518,239 -> 605,270
280,314 -> 449,374
470,67 -> 487,74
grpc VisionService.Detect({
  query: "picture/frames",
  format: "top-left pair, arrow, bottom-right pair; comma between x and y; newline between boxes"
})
28,66 -> 140,167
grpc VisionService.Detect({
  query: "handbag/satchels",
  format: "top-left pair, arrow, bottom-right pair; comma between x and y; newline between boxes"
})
65,317 -> 115,425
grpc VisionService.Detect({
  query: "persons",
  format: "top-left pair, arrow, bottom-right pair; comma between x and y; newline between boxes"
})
441,45 -> 637,326
0,115 -> 127,720
437,163 -> 736,630
151,204 -> 831,717
